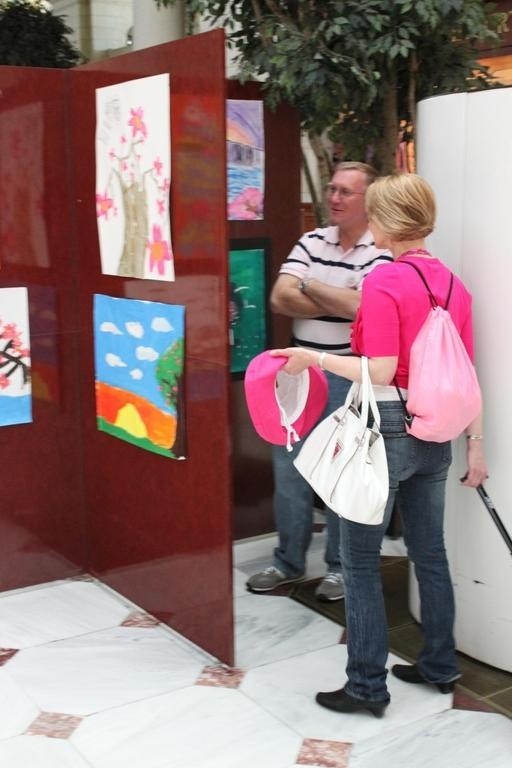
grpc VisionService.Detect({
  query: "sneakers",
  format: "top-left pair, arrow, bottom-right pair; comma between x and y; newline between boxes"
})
246,566 -> 307,592
314,571 -> 346,602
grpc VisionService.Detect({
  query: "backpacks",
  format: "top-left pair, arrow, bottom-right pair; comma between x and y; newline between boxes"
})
393,258 -> 484,445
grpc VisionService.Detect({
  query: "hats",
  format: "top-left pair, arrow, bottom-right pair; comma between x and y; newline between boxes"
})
243,349 -> 330,452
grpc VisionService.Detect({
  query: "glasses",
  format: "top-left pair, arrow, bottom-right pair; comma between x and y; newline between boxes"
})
326,184 -> 367,196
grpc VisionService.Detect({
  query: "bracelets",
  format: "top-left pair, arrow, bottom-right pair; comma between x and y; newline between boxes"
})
466,434 -> 482,440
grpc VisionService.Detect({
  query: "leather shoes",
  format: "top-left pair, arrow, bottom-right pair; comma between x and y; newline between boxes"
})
315,687 -> 390,718
393,664 -> 463,694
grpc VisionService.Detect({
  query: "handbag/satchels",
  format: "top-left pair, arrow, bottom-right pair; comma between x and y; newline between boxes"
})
292,354 -> 390,527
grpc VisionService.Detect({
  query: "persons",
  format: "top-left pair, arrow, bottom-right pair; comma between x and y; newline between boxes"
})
246,162 -> 392,603
266,172 -> 491,721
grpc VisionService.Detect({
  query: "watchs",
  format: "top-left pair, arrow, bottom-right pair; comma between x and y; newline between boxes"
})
317,352 -> 325,371
298,277 -> 313,290
